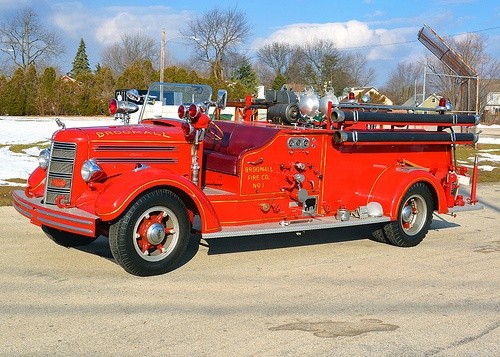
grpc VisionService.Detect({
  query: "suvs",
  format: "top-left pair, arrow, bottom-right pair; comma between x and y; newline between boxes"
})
10,82 -> 480,277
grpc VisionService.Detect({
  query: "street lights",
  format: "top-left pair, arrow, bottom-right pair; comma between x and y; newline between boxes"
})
160,27 -> 201,103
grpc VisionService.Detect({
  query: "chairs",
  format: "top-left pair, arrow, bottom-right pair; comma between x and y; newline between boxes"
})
203,120 -> 240,176
205,125 -> 283,176
